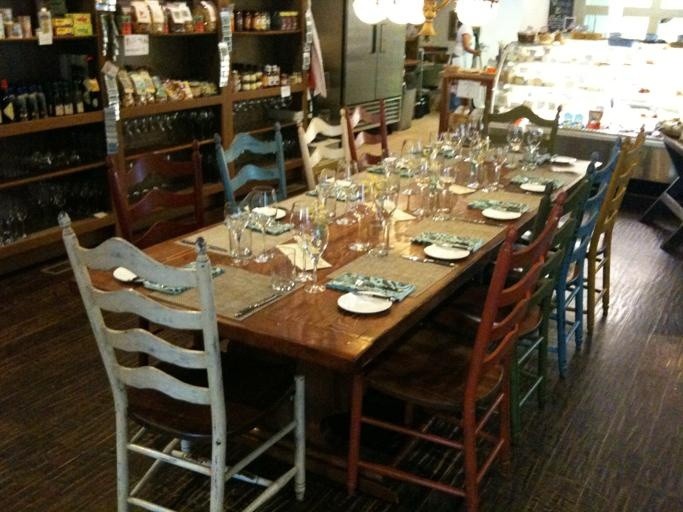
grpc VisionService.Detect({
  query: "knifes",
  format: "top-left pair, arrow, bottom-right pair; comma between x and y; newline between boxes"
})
179,240 -> 226,253
353,290 -> 396,303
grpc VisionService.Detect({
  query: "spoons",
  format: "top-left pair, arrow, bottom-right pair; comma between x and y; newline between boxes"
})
233,280 -> 293,317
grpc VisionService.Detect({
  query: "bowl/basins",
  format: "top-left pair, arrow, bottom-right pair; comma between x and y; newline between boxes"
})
517,29 -> 535,42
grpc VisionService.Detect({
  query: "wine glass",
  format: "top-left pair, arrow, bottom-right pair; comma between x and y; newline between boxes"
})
319,118 -> 578,269
222,203 -> 251,267
0,173 -> 107,244
291,199 -> 326,296
248,180 -> 276,263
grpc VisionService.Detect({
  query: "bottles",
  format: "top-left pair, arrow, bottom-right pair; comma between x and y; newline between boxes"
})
231,7 -> 298,30
120,93 -> 292,152
237,62 -> 304,90
0,76 -> 101,125
37,6 -> 55,37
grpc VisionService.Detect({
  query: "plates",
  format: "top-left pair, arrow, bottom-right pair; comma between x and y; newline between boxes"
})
113,266 -> 151,288
335,290 -> 392,315
247,206 -> 286,219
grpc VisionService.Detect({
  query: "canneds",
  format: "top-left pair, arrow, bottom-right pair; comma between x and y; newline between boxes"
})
279,11 -> 298,30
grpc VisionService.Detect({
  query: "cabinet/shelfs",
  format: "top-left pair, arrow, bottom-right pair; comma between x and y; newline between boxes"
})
0,0 -> 310,274
482,39 -> 683,208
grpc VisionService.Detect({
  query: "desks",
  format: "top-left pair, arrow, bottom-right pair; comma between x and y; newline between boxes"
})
436,66 -> 497,140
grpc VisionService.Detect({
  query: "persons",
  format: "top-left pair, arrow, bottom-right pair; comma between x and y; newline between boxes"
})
447,27 -> 482,113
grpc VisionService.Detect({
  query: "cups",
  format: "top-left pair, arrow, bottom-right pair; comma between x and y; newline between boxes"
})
226,209 -> 251,261
268,246 -> 298,292
